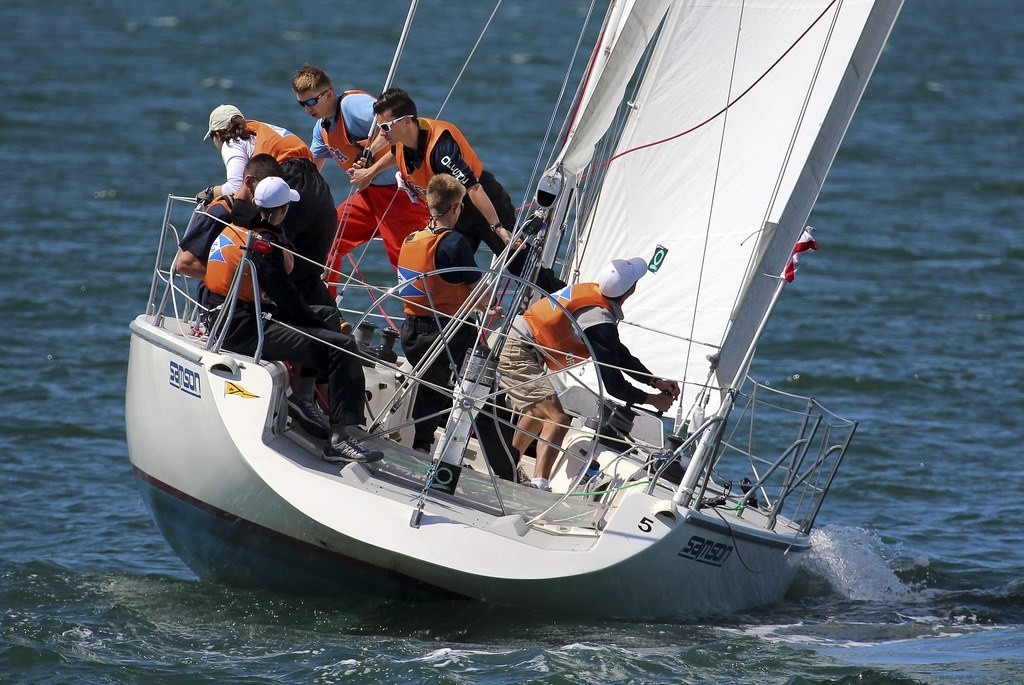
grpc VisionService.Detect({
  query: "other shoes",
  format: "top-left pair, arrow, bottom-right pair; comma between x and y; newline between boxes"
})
529,483 -> 552,492
515,463 -> 531,487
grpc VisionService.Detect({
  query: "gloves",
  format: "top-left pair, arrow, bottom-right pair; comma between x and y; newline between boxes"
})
196,185 -> 215,206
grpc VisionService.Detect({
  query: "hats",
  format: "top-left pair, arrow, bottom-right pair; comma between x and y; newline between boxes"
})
254,177 -> 300,208
203,104 -> 245,141
599,257 -> 647,297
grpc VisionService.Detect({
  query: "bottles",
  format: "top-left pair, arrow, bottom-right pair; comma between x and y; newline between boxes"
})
578,460 -> 600,485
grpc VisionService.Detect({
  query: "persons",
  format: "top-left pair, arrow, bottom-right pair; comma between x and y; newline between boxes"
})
195,104 -> 343,364
203,176 -> 385,465
397,173 -> 529,485
486,257 -> 681,493
176,153 -> 283,323
350,89 -> 568,297
293,64 -> 428,301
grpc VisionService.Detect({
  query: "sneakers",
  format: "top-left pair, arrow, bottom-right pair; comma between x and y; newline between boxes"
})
286,393 -> 332,440
321,434 -> 384,463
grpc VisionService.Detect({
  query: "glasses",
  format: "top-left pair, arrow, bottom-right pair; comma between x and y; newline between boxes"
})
376,115 -> 413,132
451,202 -> 465,213
297,87 -> 334,108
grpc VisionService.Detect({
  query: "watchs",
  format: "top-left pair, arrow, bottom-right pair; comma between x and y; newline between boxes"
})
486,297 -> 498,308
490,223 -> 502,231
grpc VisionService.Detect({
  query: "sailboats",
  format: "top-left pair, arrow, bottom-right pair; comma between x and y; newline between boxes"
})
126,0 -> 906,623
